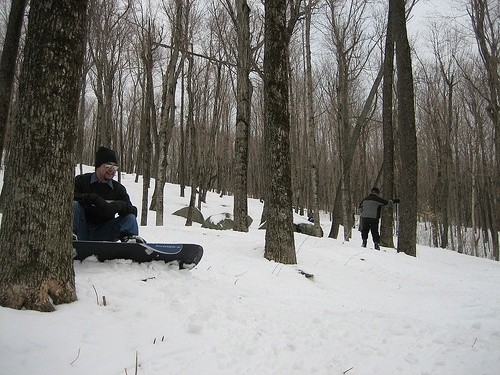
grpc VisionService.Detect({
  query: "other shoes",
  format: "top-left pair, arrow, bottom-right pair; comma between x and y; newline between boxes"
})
72,234 -> 78,241
361,239 -> 367,247
120,235 -> 147,243
375,242 -> 380,250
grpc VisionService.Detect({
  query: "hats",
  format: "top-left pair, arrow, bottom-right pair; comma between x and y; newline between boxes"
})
95,146 -> 117,170
371,188 -> 380,194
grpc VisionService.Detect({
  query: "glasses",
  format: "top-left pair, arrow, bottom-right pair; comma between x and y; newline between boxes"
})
102,163 -> 119,171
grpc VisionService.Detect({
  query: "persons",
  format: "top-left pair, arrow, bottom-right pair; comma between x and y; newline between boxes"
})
359,188 -> 400,250
71,147 -> 146,243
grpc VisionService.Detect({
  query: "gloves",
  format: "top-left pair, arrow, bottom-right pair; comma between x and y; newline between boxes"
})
102,200 -> 127,221
391,199 -> 400,204
80,193 -> 107,212
